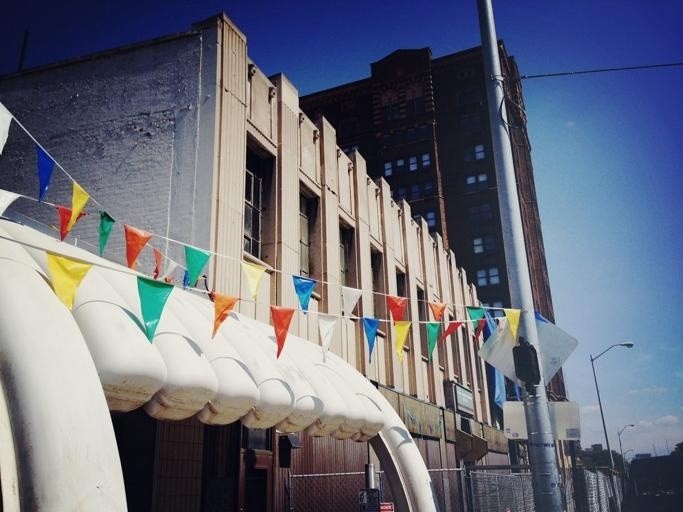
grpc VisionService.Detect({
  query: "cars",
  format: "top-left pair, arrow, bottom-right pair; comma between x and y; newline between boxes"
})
640,487 -> 665,496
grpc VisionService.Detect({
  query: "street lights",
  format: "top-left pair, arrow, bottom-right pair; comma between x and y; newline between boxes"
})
617,425 -> 634,500
624,449 -> 633,479
589,343 -> 635,512
